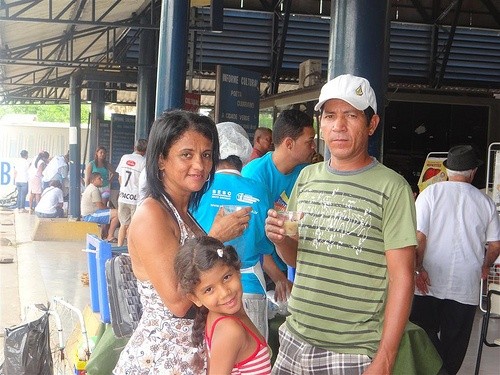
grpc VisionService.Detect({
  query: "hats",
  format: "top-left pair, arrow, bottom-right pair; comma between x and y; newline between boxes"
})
314,74 -> 377,114
443,145 -> 483,171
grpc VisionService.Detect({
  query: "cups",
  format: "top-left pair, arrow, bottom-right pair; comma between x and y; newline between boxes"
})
223,205 -> 251,216
277,210 -> 300,236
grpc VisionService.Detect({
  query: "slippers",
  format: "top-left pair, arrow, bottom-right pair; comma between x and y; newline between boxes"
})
107,237 -> 118,243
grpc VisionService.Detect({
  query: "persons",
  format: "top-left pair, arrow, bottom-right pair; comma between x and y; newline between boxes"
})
13,109 -> 324,345
111,109 -> 253,375
175,236 -> 271,375
409,145 -> 500,375
265,74 -> 416,375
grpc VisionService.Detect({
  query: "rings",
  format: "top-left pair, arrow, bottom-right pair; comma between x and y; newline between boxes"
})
243,223 -> 247,230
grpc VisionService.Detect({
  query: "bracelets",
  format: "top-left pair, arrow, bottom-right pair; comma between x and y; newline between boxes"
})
414,266 -> 425,275
